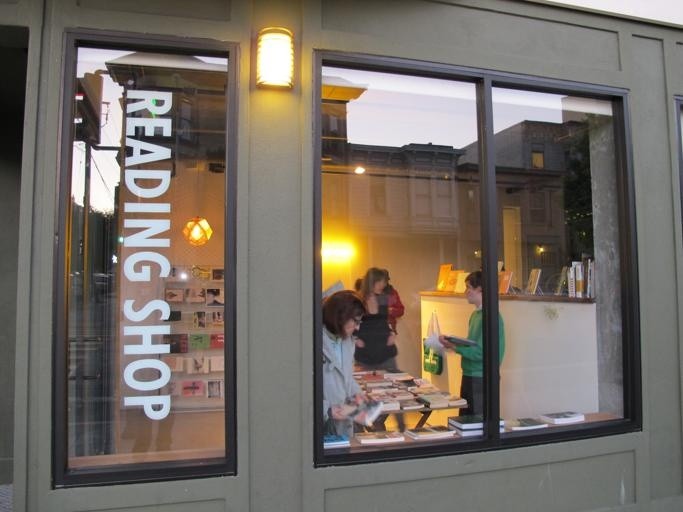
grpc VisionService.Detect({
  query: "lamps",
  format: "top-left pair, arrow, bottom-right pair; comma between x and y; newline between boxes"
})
254,26 -> 295,92
181,216 -> 213,246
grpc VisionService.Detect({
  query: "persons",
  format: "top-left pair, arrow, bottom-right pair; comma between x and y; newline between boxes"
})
353,268 -> 397,370
321,290 -> 376,437
379,268 -> 404,335
438,270 -> 504,417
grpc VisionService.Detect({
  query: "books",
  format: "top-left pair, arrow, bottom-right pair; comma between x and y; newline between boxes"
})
354,432 -> 404,445
406,433 -> 453,440
324,358 -> 468,410
506,418 -> 550,431
554,259 -> 597,300
446,335 -> 476,346
164,265 -> 225,400
544,410 -> 585,425
448,414 -> 504,431
446,425 -> 505,437
435,263 -> 470,293
405,425 -> 456,438
323,432 -> 348,445
323,442 -> 350,448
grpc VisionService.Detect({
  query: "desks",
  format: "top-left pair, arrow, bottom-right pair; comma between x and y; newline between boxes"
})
351,360 -> 469,430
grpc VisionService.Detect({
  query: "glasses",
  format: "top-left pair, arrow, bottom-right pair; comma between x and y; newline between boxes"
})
351,317 -> 363,327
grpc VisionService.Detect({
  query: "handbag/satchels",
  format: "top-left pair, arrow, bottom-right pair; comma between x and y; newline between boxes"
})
423,313 -> 444,375
